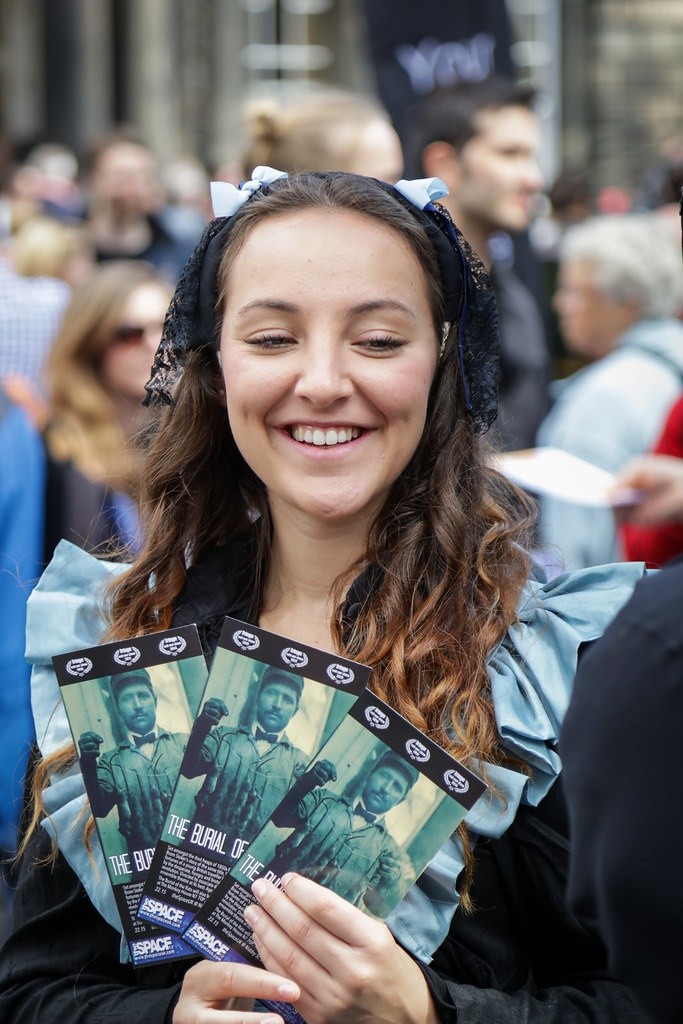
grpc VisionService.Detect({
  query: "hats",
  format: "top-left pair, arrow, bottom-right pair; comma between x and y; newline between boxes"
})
379,750 -> 420,788
262,665 -> 304,695
111,669 -> 150,700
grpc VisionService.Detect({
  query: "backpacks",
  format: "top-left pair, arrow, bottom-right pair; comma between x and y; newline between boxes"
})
610,343 -> 683,566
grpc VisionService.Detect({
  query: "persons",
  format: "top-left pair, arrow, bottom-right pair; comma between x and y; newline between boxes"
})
180,668 -> 309,866
271,752 -> 419,919
0,68 -> 683,1024
77,667 -> 192,882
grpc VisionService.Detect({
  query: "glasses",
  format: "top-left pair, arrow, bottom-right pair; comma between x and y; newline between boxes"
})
98,324 -> 162,351
